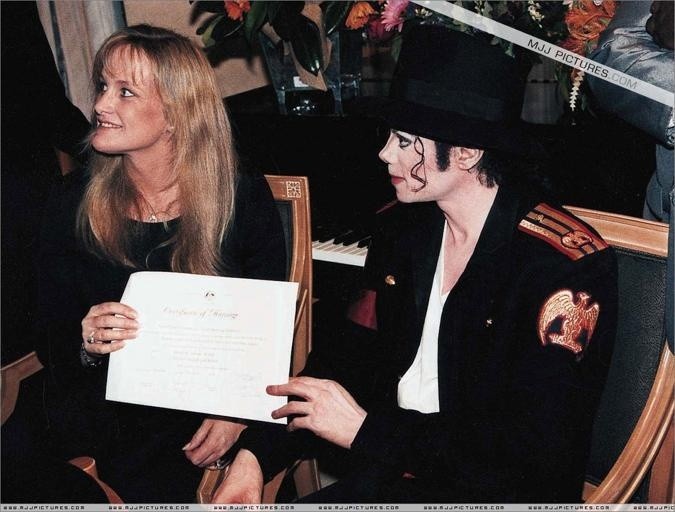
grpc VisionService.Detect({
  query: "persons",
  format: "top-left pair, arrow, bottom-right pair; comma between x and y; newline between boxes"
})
1,22 -> 287,506
585,1 -> 675,224
210,24 -> 619,506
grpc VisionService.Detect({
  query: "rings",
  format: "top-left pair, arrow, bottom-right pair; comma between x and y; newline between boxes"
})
87,330 -> 96,344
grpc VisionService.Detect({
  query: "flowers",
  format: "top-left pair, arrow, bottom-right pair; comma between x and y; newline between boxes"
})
381,1 -> 616,112
189,0 -> 385,76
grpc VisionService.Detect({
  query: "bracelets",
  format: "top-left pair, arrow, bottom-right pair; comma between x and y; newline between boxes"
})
80,343 -> 103,369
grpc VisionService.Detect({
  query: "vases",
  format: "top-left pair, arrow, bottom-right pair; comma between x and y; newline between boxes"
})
260,28 -> 362,119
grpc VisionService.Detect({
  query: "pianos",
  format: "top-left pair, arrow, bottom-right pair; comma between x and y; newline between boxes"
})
308,184 -> 398,269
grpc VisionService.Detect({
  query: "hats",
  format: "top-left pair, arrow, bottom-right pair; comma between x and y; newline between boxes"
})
340,24 -> 529,154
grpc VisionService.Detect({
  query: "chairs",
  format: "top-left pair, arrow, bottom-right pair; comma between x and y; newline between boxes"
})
291,205 -> 675,503
1,174 -> 321,504
